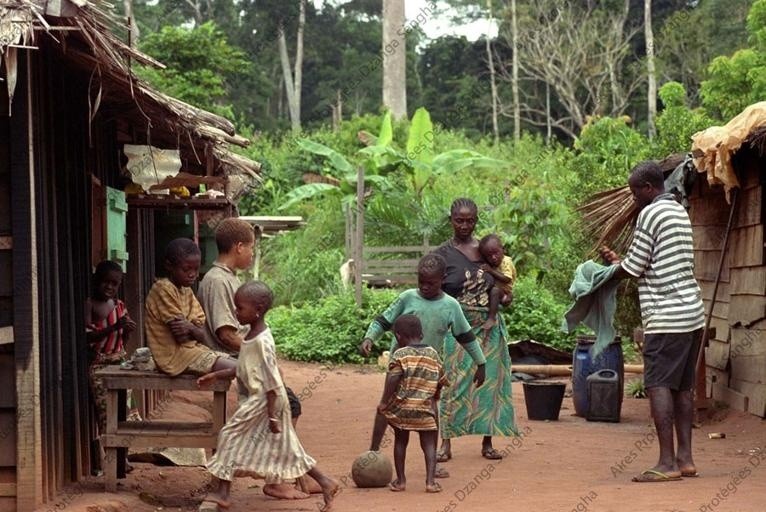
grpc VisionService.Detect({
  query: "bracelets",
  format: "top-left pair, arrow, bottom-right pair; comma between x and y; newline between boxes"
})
268,417 -> 279,422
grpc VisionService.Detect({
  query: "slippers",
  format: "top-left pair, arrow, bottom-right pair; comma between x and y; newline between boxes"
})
631,467 -> 699,482
389,448 -> 502,493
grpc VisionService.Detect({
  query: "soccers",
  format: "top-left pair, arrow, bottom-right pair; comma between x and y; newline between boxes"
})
352,450 -> 393,487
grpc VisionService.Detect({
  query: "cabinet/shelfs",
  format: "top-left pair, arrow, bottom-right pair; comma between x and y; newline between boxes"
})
125,197 -> 241,358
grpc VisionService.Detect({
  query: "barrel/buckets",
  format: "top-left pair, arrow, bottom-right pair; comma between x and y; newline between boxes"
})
571,334 -> 625,417
585,369 -> 620,423
522,380 -> 566,421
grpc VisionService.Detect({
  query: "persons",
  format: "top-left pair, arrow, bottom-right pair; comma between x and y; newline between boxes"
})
477,235 -> 516,330
432,198 -> 518,459
189,285 -> 343,511
196,215 -> 301,429
143,237 -> 238,375
376,315 -> 450,494
359,253 -> 487,478
605,162 -> 705,481
80,259 -> 136,473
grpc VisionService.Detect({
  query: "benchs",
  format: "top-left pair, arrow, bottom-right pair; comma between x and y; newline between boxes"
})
98,364 -> 231,492
